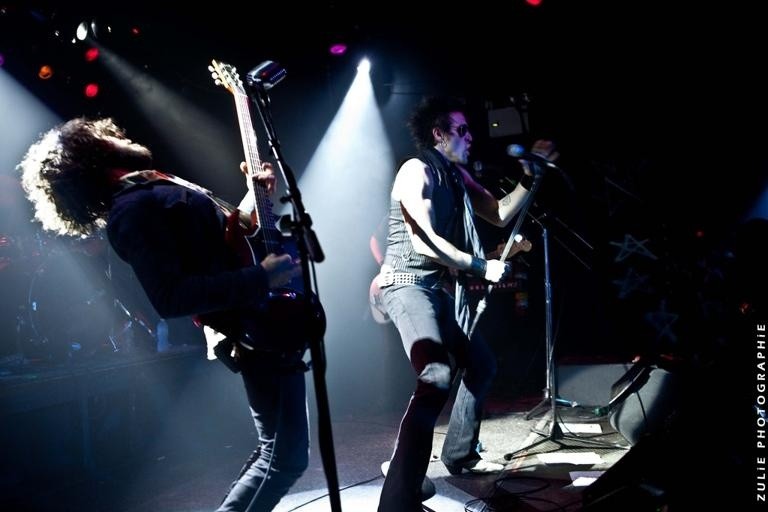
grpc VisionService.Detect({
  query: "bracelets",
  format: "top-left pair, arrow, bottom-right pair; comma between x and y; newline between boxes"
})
519,173 -> 534,192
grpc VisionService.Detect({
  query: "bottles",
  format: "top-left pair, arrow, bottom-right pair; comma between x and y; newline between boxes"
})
157,318 -> 169,353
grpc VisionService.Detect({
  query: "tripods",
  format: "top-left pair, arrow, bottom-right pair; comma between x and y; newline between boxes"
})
102,237 -> 156,353
497,185 -> 627,461
501,175 -> 593,421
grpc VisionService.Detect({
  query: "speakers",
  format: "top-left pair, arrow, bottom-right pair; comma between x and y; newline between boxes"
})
608,367 -> 678,448
555,364 -> 635,407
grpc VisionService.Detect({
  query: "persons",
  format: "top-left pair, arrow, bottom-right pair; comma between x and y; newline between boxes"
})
372,92 -> 558,511
16,113 -> 317,512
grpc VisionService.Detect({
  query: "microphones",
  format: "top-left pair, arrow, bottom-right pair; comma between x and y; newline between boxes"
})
246,59 -> 287,91
507,144 -> 560,173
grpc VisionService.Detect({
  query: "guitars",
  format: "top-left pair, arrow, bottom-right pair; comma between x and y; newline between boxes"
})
370,234 -> 534,324
208,60 -> 325,363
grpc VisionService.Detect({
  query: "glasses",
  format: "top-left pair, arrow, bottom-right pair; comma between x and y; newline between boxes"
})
451,124 -> 469,138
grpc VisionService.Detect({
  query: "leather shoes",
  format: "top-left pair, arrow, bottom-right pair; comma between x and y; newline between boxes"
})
445,458 -> 504,476
